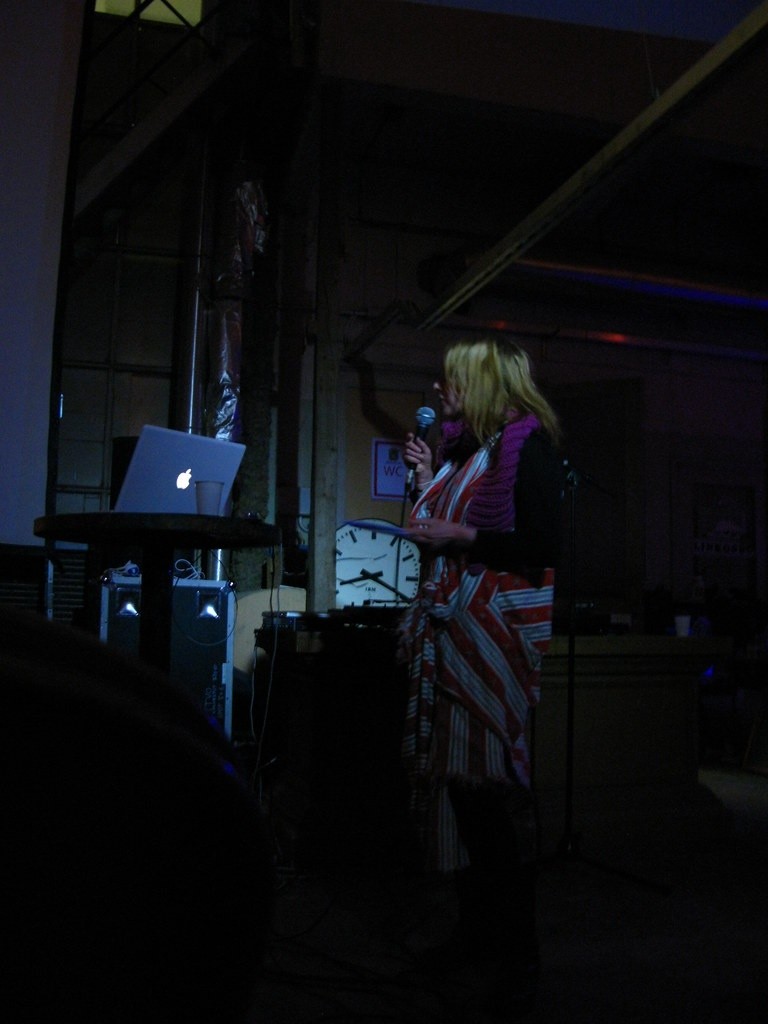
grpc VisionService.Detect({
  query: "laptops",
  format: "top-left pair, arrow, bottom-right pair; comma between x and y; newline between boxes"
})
112,425 -> 246,515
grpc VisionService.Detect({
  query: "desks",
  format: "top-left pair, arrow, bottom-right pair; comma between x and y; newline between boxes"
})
34,511 -> 270,683
525,635 -> 700,846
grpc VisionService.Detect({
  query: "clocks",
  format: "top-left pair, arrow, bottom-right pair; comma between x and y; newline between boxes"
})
335,518 -> 421,606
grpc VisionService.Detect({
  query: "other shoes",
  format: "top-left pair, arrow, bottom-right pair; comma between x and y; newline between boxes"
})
412,945 -> 461,970
502,954 -> 540,1015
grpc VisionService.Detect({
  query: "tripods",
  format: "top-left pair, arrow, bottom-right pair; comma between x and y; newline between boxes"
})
536,484 -> 674,899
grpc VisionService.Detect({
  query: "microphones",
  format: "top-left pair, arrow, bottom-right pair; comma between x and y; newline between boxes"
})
563,461 -> 600,487
406,407 -> 436,491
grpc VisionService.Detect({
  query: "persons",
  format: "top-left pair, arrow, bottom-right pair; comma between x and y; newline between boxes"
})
397,337 -> 571,1021
